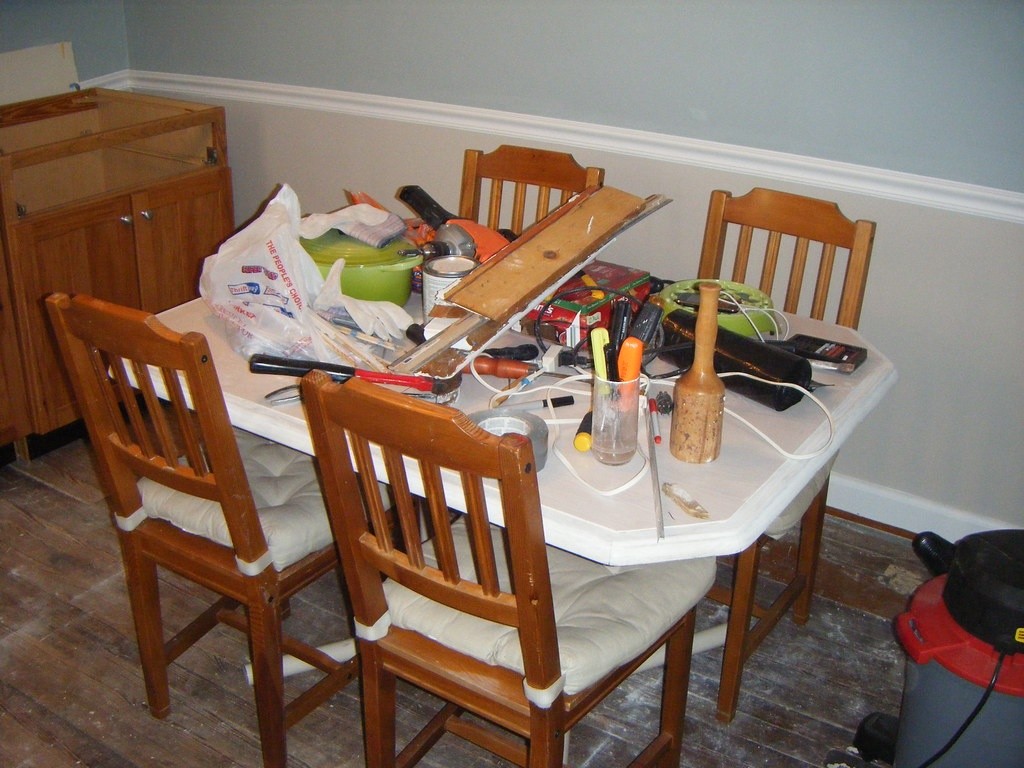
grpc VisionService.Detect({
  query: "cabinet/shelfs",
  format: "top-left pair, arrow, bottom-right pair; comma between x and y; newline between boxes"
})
0,86 -> 236,466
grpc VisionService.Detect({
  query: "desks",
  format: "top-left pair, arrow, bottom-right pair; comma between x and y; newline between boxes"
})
107,290 -> 897,723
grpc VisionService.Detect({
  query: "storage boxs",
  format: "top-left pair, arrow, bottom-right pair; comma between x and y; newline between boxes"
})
787,333 -> 867,374
510,260 -> 651,351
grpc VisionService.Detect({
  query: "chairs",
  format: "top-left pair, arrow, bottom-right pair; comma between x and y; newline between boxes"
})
697,187 -> 876,723
459,145 -> 604,235
48,293 -> 420,768
303,369 -> 717,768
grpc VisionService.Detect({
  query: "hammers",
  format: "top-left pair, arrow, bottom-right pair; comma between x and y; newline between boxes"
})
249,352 -> 463,394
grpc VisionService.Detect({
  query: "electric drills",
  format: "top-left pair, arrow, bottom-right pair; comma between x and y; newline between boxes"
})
398,182 -> 518,264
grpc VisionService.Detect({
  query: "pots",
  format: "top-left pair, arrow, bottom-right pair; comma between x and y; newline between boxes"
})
298,228 -> 423,306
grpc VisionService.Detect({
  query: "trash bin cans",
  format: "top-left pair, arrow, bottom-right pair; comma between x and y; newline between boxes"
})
852,529 -> 1023,767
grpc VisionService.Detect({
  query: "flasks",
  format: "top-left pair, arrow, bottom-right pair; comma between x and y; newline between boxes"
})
655,309 -> 812,411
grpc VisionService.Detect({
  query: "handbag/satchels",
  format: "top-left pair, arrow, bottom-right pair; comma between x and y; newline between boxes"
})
199,183 -> 373,376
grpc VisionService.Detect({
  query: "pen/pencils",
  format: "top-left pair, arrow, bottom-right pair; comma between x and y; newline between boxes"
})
649,398 -> 662,443
493,395 -> 575,411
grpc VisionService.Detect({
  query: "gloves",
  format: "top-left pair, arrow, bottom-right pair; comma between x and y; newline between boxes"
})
296,202 -> 409,250
313,258 -> 414,342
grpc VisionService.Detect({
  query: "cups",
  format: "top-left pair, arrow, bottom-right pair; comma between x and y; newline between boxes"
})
592,374 -> 640,465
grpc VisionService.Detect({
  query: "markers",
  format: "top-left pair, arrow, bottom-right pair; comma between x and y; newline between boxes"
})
603,343 -> 621,401
